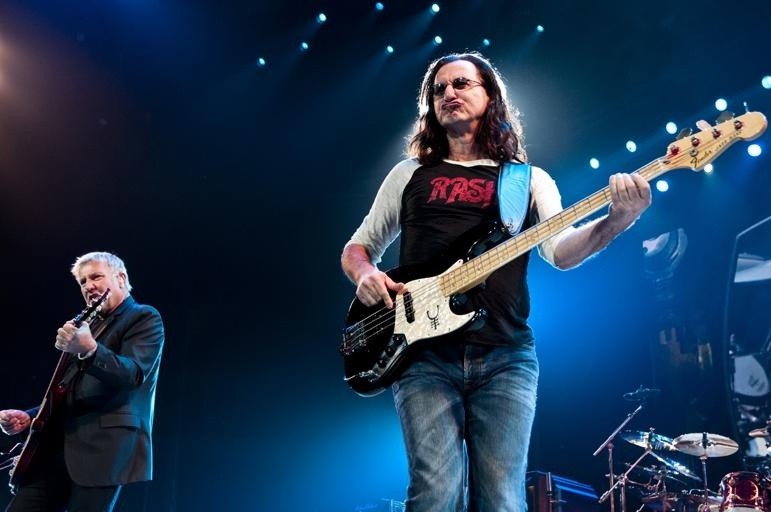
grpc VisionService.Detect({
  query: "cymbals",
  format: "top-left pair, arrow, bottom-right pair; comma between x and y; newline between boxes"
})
620,428 -> 675,450
674,433 -> 740,459
650,453 -> 703,483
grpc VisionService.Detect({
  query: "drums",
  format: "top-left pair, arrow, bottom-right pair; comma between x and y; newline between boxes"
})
717,472 -> 768,511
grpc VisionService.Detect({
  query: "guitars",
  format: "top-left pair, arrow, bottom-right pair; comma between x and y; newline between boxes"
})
338,99 -> 769,397
8,288 -> 109,492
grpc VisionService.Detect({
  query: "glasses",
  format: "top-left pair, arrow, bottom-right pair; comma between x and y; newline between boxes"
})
430,76 -> 485,98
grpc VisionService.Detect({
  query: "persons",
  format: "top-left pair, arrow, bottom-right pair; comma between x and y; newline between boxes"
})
0,251 -> 167,512
340,54 -> 653,511
